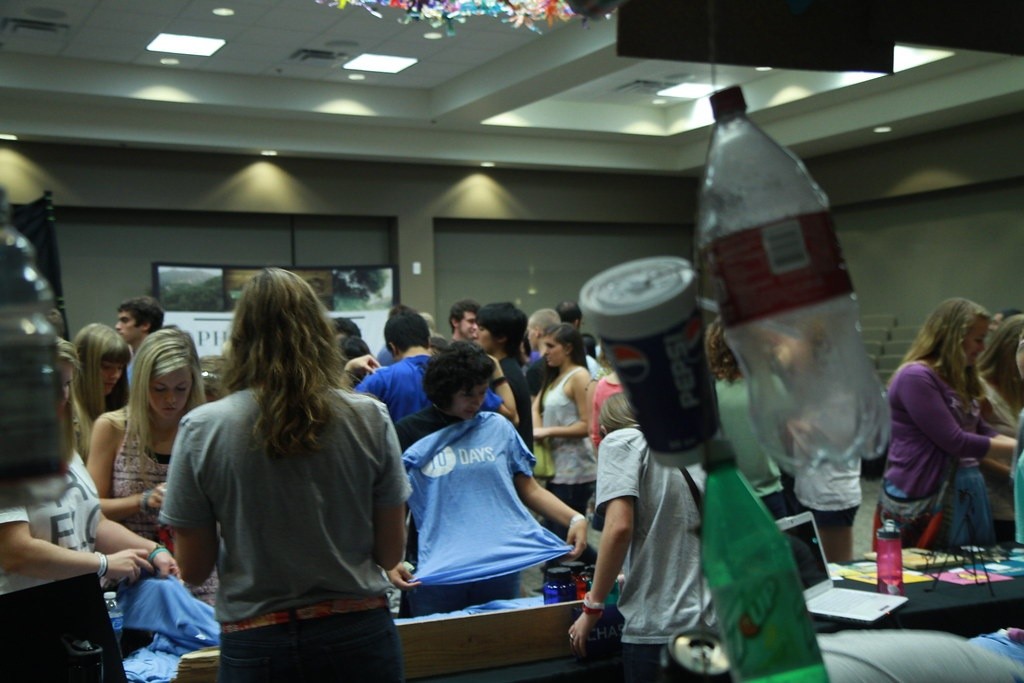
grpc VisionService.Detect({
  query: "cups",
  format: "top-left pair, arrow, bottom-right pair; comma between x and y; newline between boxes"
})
576,254 -> 720,465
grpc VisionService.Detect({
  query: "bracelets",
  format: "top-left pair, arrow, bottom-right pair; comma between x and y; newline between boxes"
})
139,487 -> 155,517
95,552 -> 108,578
583,590 -> 606,619
147,545 -> 170,563
568,514 -> 586,524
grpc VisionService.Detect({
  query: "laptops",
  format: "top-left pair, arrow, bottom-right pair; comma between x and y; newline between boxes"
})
774,511 -> 909,624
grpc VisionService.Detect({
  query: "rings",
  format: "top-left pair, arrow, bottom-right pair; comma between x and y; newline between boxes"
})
569,633 -> 574,640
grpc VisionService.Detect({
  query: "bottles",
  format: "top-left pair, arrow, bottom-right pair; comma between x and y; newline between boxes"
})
876,520 -> 904,595
699,438 -> 831,683
104,593 -> 123,658
700,83 -> 892,468
542,562 -> 620,605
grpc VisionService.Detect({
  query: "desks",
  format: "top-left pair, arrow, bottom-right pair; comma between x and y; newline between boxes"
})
411,542 -> 1023,683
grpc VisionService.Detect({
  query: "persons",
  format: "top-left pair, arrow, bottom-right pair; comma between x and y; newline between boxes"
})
326,299 -> 729,683
871,297 -> 1024,551
0,306 -> 183,683
57,295 -> 232,609
158,269 -> 414,683
707,311 -> 862,562
